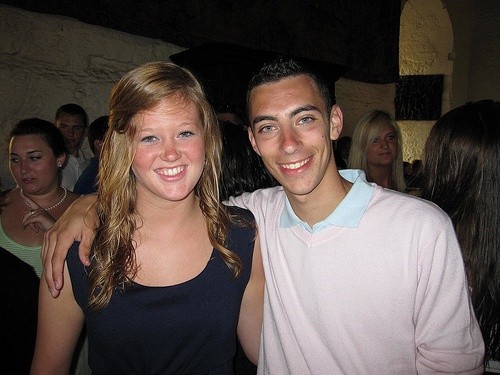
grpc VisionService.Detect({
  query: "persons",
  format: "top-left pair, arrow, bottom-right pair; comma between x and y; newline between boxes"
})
347,109 -> 406,196
72,115 -> 112,194
53,104 -> 94,193
0,117 -> 95,375
209,107 -> 349,201
0,245 -> 40,375
40,54 -> 487,375
31,59 -> 266,375
417,93 -> 500,375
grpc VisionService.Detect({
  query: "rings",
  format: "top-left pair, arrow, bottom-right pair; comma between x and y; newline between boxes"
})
32,209 -> 35,214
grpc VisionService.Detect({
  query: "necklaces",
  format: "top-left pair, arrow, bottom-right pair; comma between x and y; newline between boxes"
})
19,184 -> 67,211
